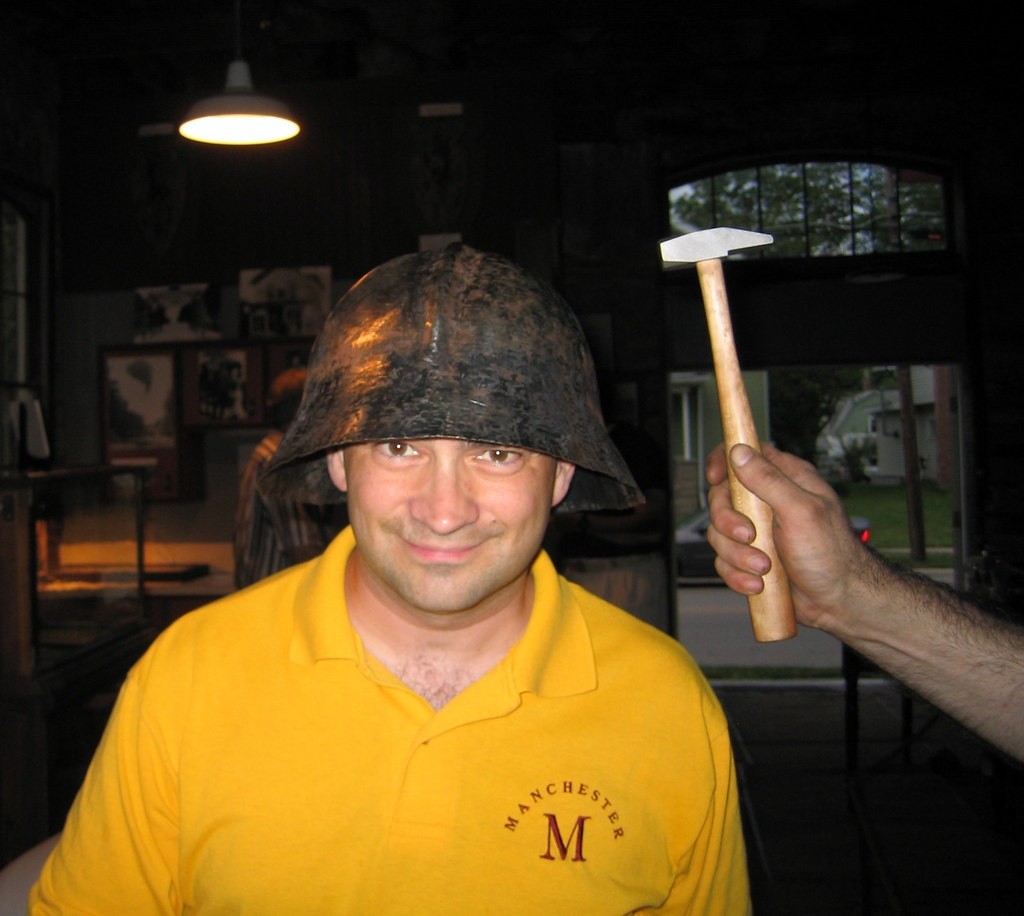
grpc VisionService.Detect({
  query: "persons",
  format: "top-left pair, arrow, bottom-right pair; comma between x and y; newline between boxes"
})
231,366 -> 346,595
27,245 -> 751,914
707,441 -> 1024,755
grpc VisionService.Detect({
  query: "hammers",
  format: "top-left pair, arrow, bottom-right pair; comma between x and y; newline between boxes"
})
660,226 -> 798,645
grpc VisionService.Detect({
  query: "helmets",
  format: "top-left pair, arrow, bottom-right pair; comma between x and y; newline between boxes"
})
256,243 -> 647,512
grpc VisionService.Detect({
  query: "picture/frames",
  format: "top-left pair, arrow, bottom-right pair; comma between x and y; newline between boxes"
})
94,333 -> 315,444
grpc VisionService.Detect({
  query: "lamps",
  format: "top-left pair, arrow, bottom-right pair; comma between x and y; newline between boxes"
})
175,0 -> 302,147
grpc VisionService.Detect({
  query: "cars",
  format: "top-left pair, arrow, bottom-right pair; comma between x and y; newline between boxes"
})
671,506 -> 870,580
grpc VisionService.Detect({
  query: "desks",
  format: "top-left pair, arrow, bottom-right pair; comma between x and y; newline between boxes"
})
25,458 -> 159,718
